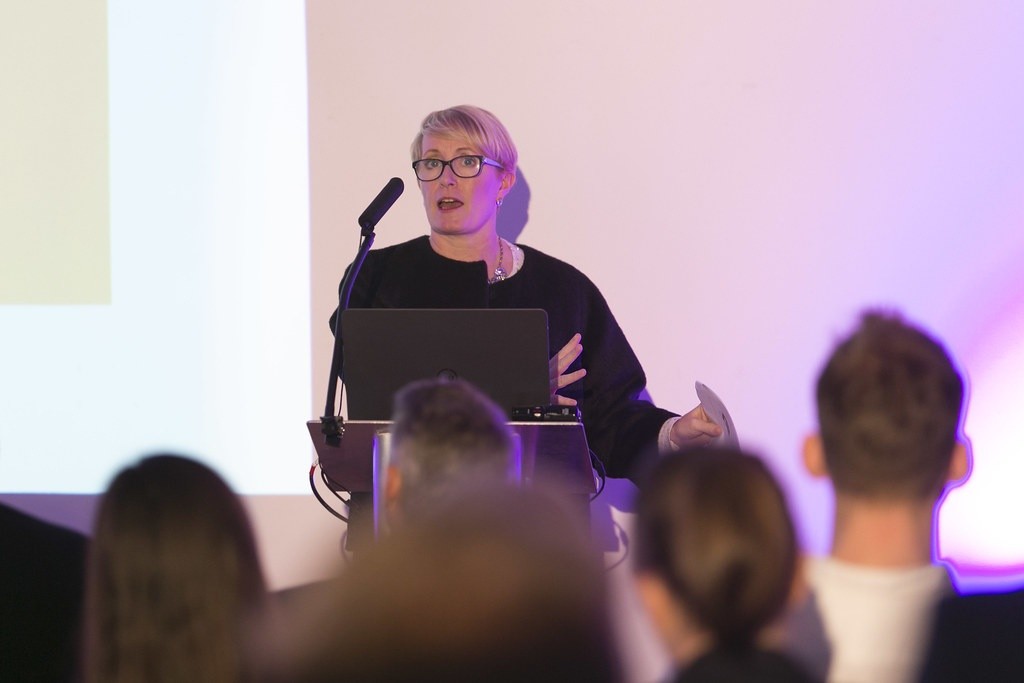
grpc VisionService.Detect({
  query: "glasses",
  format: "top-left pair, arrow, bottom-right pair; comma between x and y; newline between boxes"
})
412,155 -> 505,182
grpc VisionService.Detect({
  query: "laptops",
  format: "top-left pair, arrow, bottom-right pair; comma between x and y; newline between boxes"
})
339,308 -> 550,422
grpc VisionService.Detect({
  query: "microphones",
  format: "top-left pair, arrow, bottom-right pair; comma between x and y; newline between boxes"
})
358,177 -> 404,236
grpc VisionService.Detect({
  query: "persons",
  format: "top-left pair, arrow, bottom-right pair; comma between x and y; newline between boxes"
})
88,451 -> 272,681
376,376 -> 524,540
800,307 -> 1024,683
328,105 -> 725,501
630,444 -> 830,683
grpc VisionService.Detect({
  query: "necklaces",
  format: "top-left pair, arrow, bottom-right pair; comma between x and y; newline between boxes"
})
488,235 -> 507,286
